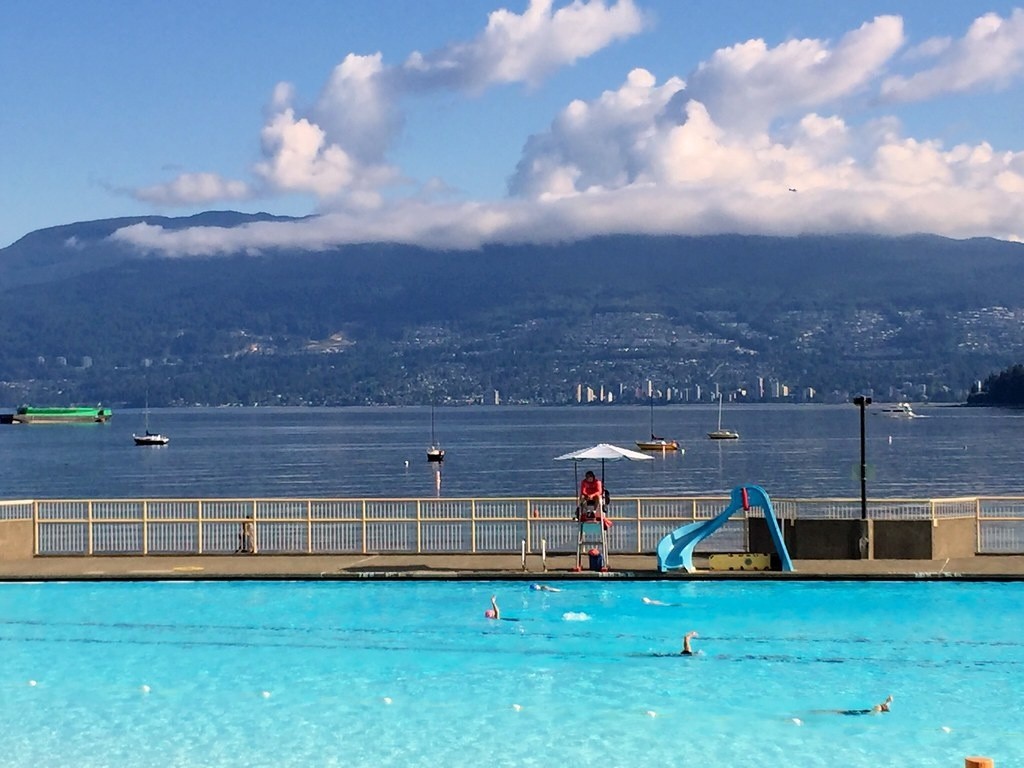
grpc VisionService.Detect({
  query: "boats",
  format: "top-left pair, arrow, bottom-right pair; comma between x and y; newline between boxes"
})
870,402 -> 915,419
0,404 -> 113,425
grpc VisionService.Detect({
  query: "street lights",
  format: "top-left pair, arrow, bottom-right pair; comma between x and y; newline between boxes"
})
852,396 -> 873,519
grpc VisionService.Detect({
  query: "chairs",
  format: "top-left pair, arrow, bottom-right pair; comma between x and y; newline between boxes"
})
579,487 -> 606,521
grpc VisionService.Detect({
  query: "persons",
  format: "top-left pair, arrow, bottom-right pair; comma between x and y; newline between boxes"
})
579,471 -> 603,517
235,515 -> 256,554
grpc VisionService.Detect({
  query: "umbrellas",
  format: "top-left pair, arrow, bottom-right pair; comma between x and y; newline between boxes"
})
553,442 -> 655,491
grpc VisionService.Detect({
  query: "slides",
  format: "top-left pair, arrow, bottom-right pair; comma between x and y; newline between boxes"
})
656,485 -> 740,572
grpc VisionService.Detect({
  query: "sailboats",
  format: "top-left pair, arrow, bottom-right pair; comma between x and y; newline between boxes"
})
132,389 -> 170,446
634,389 -> 680,451
425,399 -> 446,461
706,392 -> 740,439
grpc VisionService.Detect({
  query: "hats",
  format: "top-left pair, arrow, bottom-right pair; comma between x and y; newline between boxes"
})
642,597 -> 648,602
881,704 -> 889,711
486,610 -> 495,617
530,583 -> 538,589
